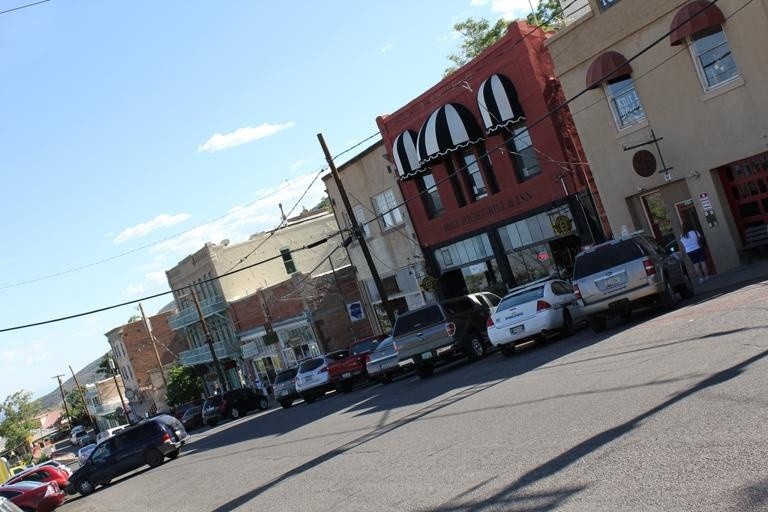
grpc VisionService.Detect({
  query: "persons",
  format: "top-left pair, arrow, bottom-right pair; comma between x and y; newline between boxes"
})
262,376 -> 274,400
680,221 -> 710,282
255,378 -> 263,394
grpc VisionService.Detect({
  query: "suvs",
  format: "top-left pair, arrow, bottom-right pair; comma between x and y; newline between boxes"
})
273,365 -> 301,408
203,388 -> 268,427
295,349 -> 349,402
73,430 -> 88,445
392,291 -> 502,378
67,414 -> 190,496
572,230 -> 694,333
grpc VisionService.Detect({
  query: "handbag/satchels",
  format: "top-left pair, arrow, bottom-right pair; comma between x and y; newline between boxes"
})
694,230 -> 706,249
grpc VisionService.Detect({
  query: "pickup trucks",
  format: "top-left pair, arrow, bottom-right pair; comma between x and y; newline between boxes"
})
328,334 -> 390,392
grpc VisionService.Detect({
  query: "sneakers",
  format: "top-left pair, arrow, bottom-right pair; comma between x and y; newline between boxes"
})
699,278 -> 704,284
705,276 -> 710,281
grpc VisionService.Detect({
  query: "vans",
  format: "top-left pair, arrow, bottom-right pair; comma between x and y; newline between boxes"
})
96,423 -> 129,444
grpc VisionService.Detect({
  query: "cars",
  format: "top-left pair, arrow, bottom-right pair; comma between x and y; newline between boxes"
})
75,443 -> 97,466
1,481 -> 65,511
366,336 -> 414,384
180,404 -> 204,429
35,460 -> 73,484
79,436 -> 95,447
486,279 -> 582,355
53,439 -> 74,453
3,467 -> 69,496
